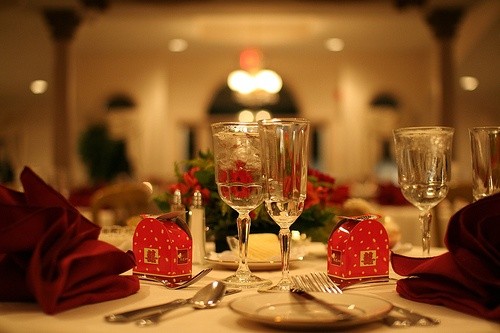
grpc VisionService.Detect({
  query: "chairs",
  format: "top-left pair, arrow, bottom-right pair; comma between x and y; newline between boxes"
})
91,187 -> 149,237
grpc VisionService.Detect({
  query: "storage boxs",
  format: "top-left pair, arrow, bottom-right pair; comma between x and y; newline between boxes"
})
327,214 -> 391,290
133,212 -> 193,289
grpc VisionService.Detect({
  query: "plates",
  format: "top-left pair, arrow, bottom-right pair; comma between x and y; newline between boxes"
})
207,256 -> 292,271
227,290 -> 412,328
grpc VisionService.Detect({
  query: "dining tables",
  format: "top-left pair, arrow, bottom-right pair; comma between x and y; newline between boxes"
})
0,234 -> 500,333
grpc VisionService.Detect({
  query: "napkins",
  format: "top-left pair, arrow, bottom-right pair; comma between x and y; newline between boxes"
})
0,166 -> 140,316
374,179 -> 414,207
389,190 -> 500,324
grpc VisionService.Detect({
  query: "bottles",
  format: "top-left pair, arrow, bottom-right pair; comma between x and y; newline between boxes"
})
171,188 -> 186,222
188,190 -> 206,266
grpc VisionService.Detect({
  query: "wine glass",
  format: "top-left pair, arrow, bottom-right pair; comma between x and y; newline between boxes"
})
211,121 -> 272,290
468,126 -> 500,203
393,126 -> 455,257
258,118 -> 311,293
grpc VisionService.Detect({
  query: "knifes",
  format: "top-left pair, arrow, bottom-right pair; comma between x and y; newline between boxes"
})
291,287 -> 353,320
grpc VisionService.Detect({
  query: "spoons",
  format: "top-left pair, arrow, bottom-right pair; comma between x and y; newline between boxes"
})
104,280 -> 226,322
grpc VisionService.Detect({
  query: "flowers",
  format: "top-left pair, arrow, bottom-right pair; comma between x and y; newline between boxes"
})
155,147 -> 352,254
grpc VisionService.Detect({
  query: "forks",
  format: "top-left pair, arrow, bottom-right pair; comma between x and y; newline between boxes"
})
293,273 -> 440,326
139,267 -> 213,291
